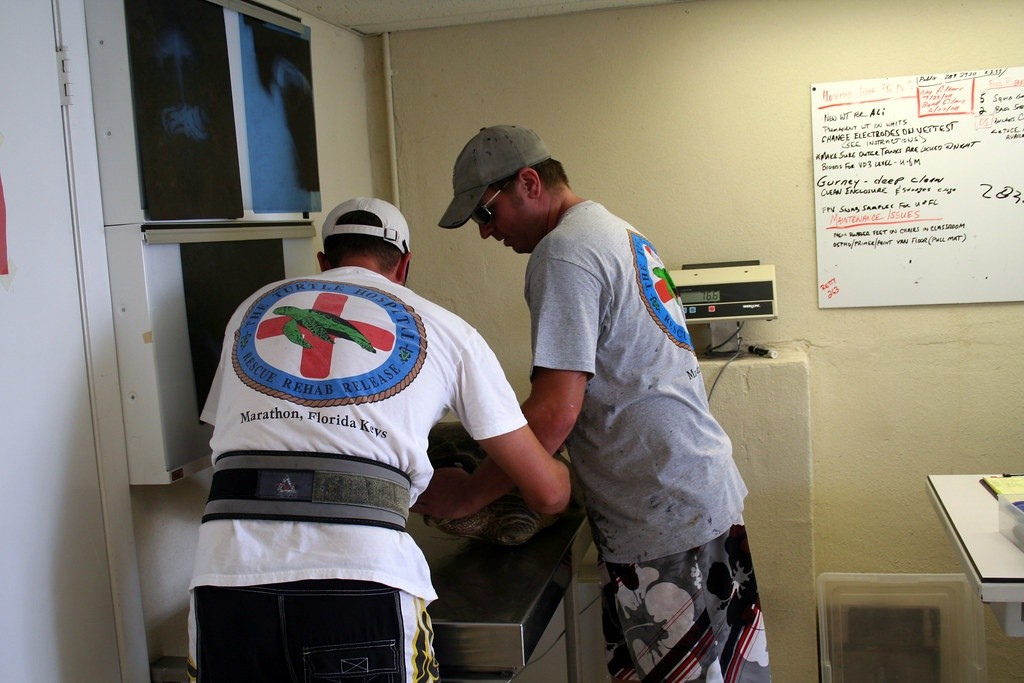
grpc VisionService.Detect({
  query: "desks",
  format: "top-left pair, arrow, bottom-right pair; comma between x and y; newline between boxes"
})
923,474 -> 1024,637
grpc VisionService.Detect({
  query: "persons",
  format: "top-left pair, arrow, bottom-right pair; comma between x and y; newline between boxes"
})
411,125 -> 777,683
187,195 -> 574,683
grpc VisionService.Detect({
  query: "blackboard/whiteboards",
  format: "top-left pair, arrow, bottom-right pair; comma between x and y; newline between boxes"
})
807,65 -> 1024,310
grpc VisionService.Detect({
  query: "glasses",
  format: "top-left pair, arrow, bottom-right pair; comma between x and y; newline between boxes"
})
471,177 -> 511,225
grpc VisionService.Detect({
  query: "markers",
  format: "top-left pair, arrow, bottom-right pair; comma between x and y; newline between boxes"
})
747,342 -> 778,359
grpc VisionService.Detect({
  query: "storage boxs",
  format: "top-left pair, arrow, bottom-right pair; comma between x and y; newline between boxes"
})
997,494 -> 1024,553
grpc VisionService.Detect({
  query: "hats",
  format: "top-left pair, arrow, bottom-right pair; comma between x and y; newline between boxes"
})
438,124 -> 551,229
322,197 -> 409,254
